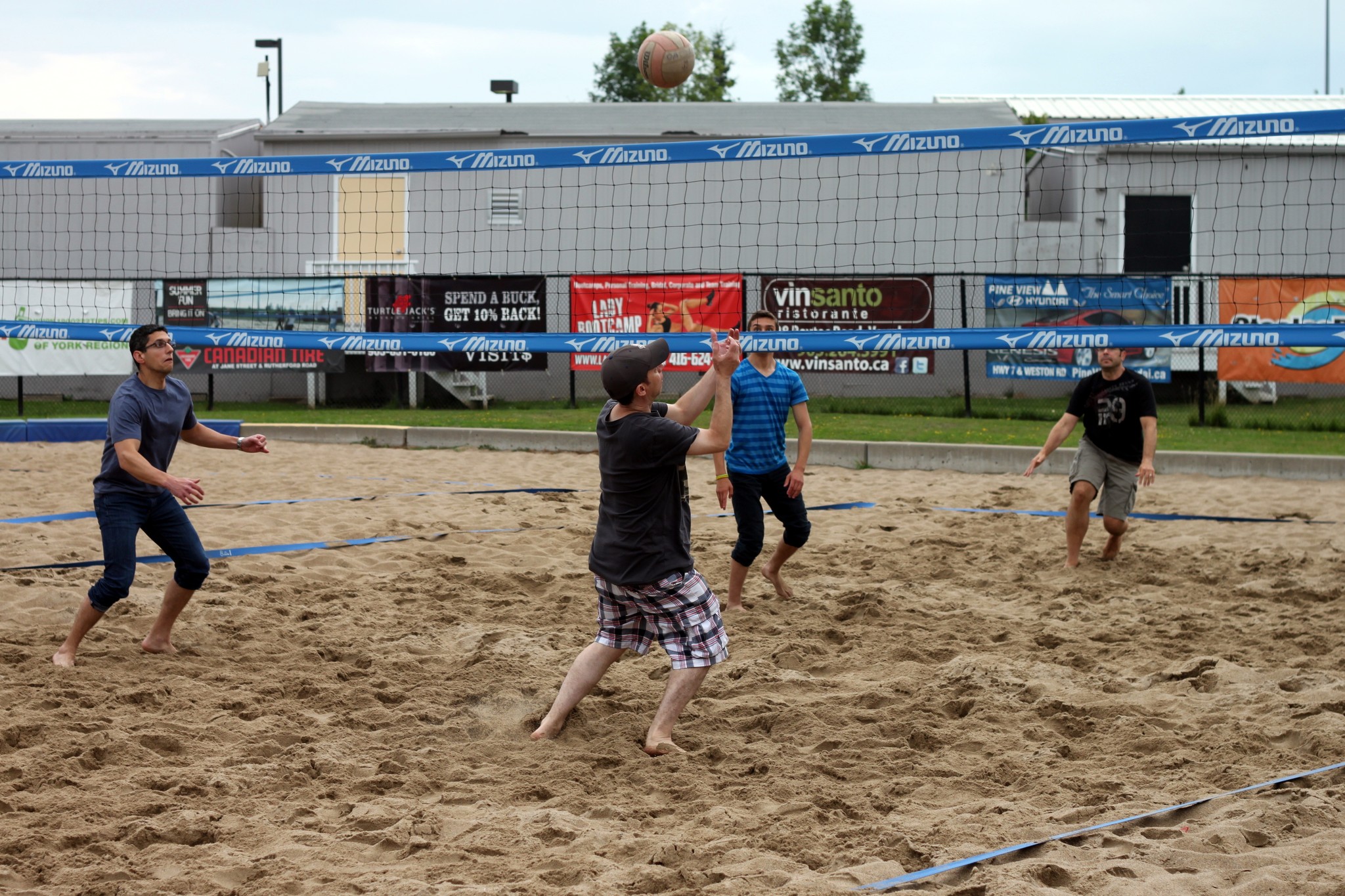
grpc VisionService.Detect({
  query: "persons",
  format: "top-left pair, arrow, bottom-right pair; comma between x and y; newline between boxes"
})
53,323 -> 269,668
528,328 -> 742,756
712,311 -> 813,614
276,311 -> 296,332
647,290 -> 716,335
1023,345 -> 1159,571
208,309 -> 222,328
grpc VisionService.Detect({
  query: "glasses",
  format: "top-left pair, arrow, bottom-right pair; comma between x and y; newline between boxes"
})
141,339 -> 176,351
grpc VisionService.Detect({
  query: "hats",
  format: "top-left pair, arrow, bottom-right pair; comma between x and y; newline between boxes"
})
601,337 -> 670,399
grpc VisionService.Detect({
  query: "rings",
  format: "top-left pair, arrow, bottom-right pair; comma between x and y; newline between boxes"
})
1149,474 -> 1152,476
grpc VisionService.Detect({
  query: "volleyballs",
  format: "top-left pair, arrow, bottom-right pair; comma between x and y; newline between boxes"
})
637,30 -> 695,89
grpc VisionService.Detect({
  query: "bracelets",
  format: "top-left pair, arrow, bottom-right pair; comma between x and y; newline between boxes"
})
237,436 -> 242,451
714,474 -> 730,481
